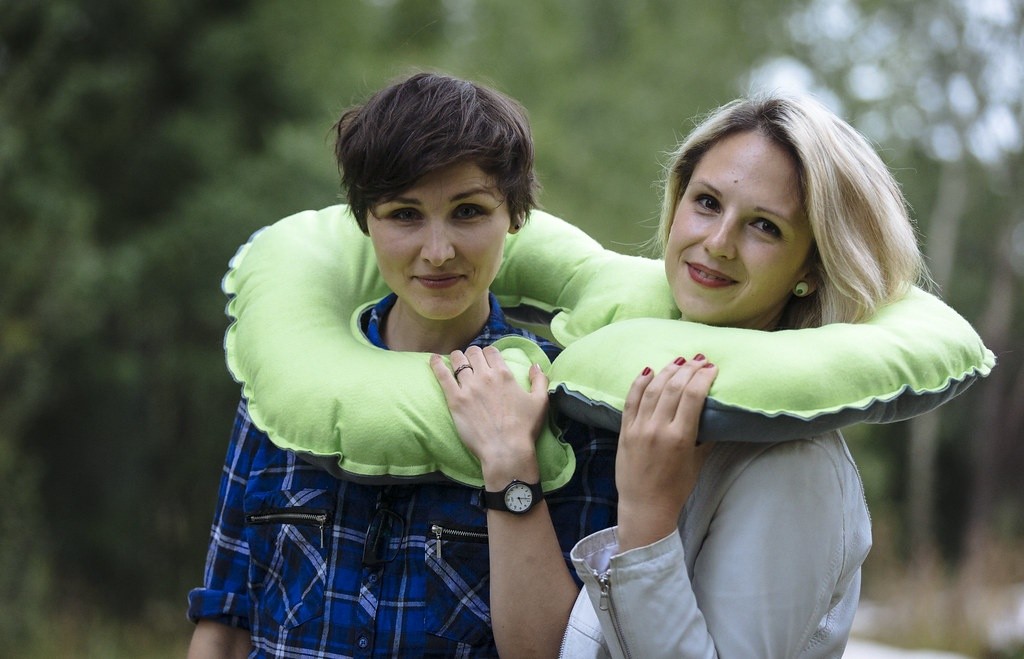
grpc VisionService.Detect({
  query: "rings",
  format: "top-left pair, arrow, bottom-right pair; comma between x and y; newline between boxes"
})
454,364 -> 473,379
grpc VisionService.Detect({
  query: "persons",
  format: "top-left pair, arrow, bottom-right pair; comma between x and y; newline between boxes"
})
185,71 -> 943,659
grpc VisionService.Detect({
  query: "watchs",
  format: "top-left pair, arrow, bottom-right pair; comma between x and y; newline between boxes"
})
478,478 -> 544,515
